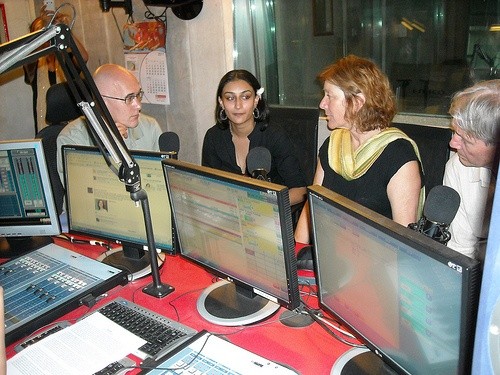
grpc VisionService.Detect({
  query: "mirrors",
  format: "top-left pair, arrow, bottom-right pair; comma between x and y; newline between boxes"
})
311,0 -> 333,36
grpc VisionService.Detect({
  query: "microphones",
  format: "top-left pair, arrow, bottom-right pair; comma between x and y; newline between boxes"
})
246,147 -> 272,182
159,131 -> 180,159
416,185 -> 462,243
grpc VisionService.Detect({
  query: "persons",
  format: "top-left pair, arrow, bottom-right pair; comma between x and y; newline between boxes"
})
199,66 -> 308,206
294,54 -> 426,245
442,78 -> 500,259
96,200 -> 107,211
54,65 -> 162,233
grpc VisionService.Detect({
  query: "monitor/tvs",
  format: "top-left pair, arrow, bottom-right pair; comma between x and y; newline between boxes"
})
0,138 -> 61,253
62,145 -> 176,282
161,158 -> 301,326
307,185 -> 481,375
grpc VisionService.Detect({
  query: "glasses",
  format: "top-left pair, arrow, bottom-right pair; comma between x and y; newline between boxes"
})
102,90 -> 144,104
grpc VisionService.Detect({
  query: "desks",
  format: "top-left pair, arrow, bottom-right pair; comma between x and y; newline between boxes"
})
0,231 -> 365,375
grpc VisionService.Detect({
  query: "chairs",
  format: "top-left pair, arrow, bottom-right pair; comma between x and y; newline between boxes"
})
33,81 -> 93,214
389,122 -> 454,223
267,106 -> 319,216
